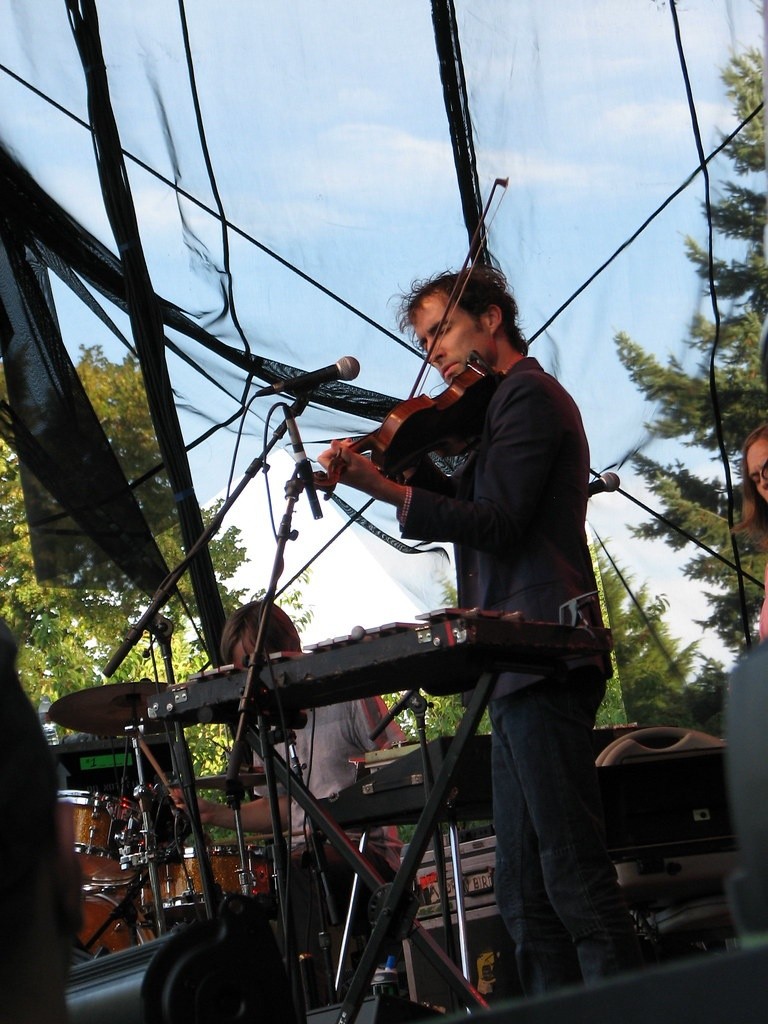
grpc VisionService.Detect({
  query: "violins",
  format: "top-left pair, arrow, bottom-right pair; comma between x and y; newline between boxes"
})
309,363 -> 501,503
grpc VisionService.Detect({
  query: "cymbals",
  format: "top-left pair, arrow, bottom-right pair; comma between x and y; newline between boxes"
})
46,676 -> 200,736
167,766 -> 282,790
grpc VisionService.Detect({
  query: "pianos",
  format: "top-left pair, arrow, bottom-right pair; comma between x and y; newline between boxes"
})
146,605 -> 617,729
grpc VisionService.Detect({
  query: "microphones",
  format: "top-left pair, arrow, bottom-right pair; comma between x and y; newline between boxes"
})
283,405 -> 322,520
255,356 -> 360,397
587,473 -> 620,498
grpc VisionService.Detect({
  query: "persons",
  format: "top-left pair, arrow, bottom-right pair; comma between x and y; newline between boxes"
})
743,422 -> 768,641
316,264 -> 645,1000
173,600 -> 407,884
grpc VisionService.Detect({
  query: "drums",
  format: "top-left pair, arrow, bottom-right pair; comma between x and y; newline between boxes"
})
75,893 -> 157,957
286,839 -> 403,981
56,788 -> 144,886
140,843 -> 272,930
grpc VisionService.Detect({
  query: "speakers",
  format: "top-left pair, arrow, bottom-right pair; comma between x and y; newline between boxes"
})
306,994 -> 446,1024
